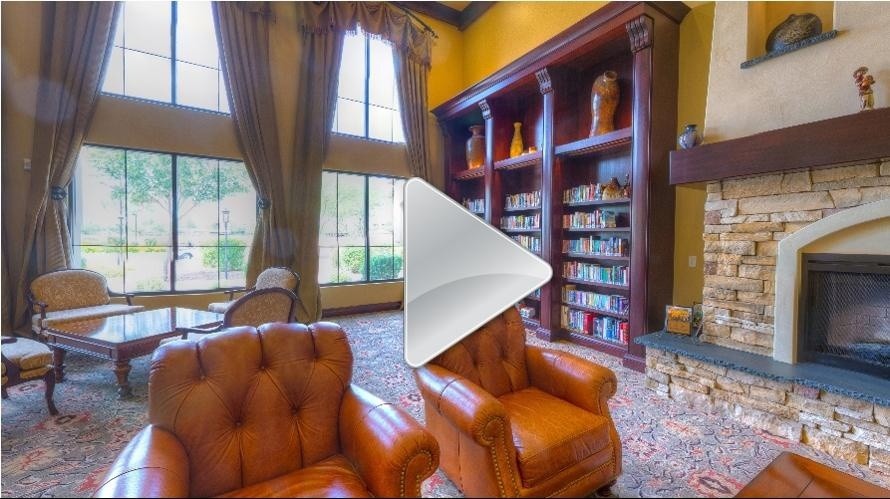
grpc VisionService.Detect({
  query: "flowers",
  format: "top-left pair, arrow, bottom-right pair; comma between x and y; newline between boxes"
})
851,64 -> 876,106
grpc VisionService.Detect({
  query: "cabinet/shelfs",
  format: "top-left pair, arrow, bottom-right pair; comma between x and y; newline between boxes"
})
429,1 -> 692,359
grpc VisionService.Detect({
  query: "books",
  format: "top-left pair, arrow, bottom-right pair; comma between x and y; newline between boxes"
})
462,182 -> 630,345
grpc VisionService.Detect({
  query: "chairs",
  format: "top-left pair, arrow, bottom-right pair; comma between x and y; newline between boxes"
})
413,305 -> 623,498
208,266 -> 301,313
25,269 -> 146,380
89,322 -> 440,498
1,336 -> 59,416
158,286 -> 297,346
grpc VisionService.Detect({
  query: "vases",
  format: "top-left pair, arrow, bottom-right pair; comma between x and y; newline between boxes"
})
857,88 -> 874,112
587,70 -> 621,138
678,124 -> 702,149
465,125 -> 485,171
509,122 -> 524,158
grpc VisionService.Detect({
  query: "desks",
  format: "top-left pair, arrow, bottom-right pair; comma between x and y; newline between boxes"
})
46,306 -> 227,397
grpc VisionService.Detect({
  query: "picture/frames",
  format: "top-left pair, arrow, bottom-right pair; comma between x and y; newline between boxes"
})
666,305 -> 694,336
